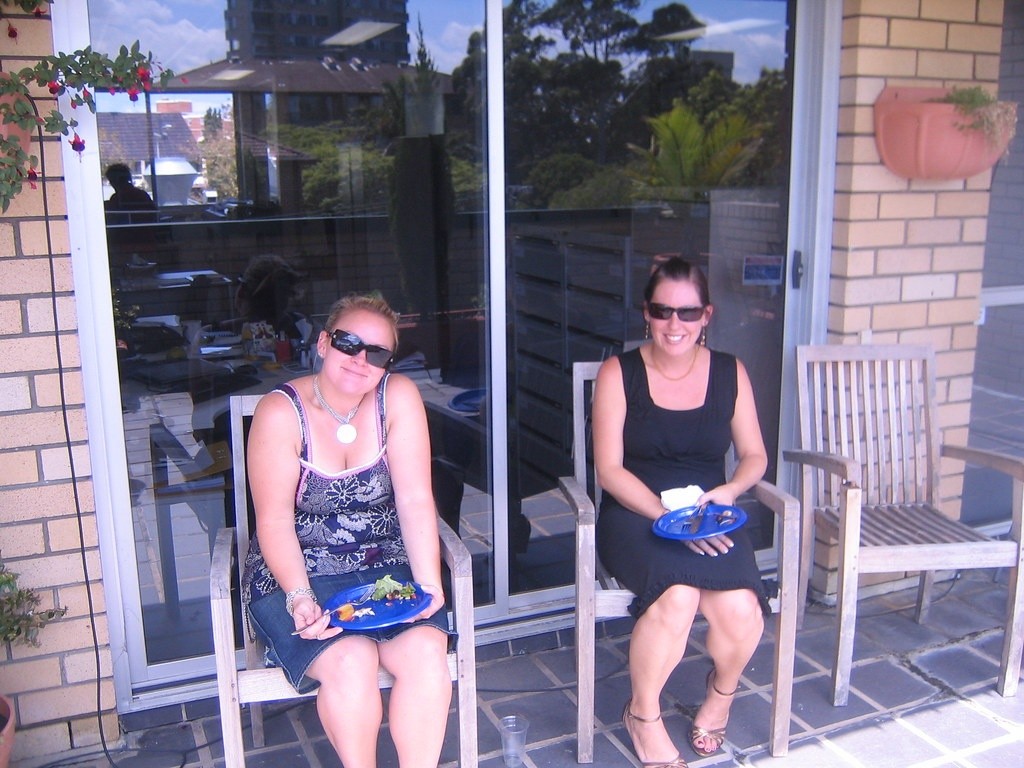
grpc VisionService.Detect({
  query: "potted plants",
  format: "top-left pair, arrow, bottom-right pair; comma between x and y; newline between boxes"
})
872,84 -> 1018,182
0,549 -> 68,768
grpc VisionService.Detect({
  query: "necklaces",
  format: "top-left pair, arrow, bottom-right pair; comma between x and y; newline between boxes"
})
314,378 -> 361,445
652,341 -> 697,381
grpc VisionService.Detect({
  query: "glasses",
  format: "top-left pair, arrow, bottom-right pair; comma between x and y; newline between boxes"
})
647,302 -> 706,322
323,327 -> 396,370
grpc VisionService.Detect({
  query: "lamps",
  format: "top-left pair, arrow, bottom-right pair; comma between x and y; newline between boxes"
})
645,0 -> 731,41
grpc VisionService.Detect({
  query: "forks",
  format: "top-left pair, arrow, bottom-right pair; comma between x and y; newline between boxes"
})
680,501 -> 701,536
291,586 -> 376,636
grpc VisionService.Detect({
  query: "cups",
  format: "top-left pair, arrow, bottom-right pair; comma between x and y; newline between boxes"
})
499,716 -> 530,767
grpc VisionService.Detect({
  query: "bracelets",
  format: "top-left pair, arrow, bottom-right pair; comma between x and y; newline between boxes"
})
286,588 -> 317,616
662,509 -> 670,516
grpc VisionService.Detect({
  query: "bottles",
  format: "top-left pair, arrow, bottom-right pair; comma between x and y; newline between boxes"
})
299,341 -> 310,369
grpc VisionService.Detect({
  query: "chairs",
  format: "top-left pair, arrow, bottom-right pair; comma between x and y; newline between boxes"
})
559,361 -> 800,768
782,342 -> 1024,708
210,394 -> 477,768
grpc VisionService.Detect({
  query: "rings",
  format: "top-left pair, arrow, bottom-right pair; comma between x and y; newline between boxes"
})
692,538 -> 697,542
317,635 -> 321,640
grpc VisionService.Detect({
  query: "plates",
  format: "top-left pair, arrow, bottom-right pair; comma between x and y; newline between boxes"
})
653,505 -> 746,540
448,387 -> 485,411
324,582 -> 432,630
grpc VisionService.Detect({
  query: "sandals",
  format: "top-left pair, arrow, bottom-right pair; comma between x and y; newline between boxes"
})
689,667 -> 740,757
622,697 -> 687,768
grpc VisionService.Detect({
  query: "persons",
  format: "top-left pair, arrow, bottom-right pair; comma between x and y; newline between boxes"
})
104,163 -> 154,244
245,296 -> 458,768
191,235 -> 599,545
591,257 -> 768,768
